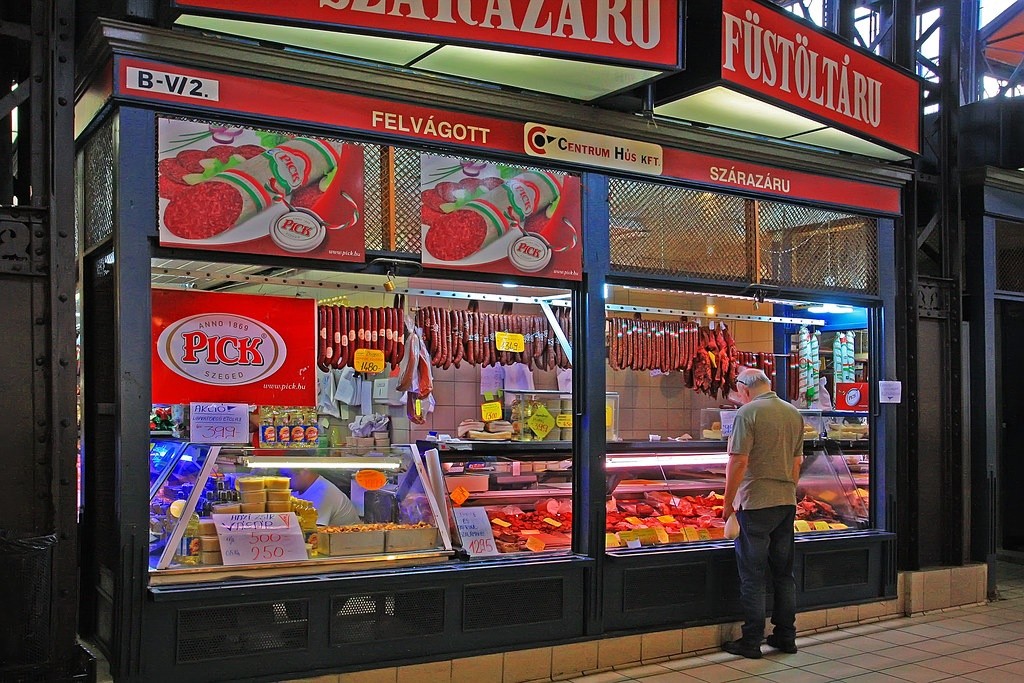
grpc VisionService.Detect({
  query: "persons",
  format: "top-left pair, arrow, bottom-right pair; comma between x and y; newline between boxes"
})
721,369 -> 804,659
275,468 -> 363,527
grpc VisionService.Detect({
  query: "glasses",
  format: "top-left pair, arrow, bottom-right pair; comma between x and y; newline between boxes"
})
734,376 -> 747,387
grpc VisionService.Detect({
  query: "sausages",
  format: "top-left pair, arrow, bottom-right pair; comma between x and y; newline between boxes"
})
317,304 -> 700,379
420,175 -> 505,262
736,351 -> 800,401
159,145 -> 268,240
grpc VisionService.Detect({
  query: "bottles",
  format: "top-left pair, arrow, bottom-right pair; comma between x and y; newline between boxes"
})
259,409 -> 319,451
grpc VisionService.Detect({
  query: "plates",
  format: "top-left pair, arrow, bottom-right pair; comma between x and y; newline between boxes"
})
419,153 -> 523,266
155,115 -> 294,245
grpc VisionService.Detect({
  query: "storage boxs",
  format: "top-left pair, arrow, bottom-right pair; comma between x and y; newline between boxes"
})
345,431 -> 390,454
385,527 -> 438,553
318,532 -> 385,557
445,474 -> 490,493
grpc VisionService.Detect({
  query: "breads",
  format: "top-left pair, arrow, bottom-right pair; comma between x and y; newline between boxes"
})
701,421 -> 869,441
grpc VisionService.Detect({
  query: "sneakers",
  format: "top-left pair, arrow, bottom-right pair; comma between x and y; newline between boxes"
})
722,638 -> 762,659
766,632 -> 798,654
